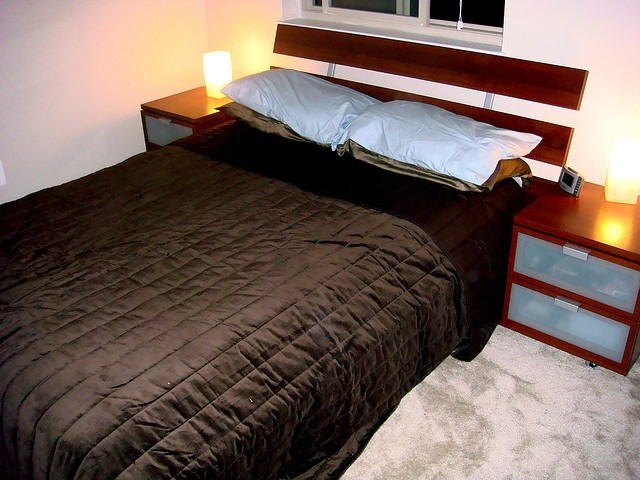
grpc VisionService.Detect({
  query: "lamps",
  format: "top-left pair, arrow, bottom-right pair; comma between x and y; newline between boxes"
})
603,140 -> 639,204
202,50 -> 234,100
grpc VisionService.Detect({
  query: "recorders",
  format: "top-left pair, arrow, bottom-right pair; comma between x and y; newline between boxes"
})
557,165 -> 586,198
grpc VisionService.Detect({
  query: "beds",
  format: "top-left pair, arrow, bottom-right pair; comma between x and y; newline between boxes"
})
0,24 -> 590,480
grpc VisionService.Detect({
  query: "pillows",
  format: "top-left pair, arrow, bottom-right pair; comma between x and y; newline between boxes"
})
221,68 -> 382,152
345,100 -> 544,188
216,102 -> 347,157
349,139 -> 532,193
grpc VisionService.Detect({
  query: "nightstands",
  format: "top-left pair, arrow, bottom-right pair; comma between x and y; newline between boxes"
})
140,83 -> 234,152
500,180 -> 640,377
